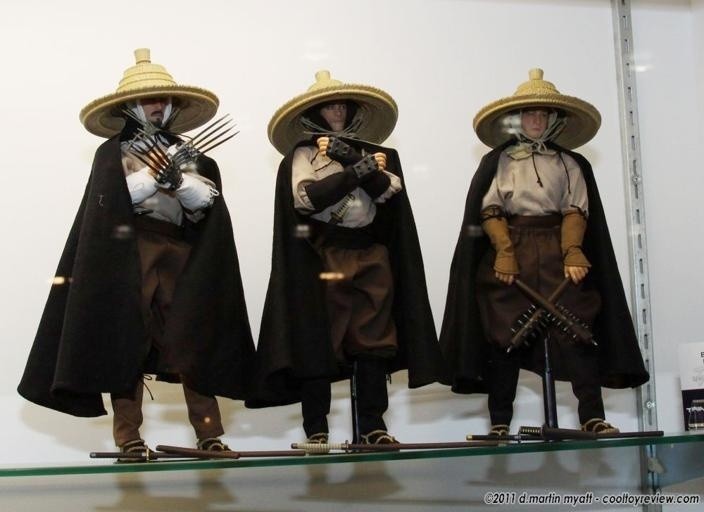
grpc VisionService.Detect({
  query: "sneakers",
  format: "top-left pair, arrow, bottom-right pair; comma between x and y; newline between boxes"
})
196,438 -> 233,459
358,430 -> 400,452
305,432 -> 328,455
488,424 -> 510,437
118,439 -> 157,461
581,419 -> 619,435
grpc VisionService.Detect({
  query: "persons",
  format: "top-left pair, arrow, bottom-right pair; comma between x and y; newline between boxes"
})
16,45 -> 258,462
440,67 -> 652,446
245,67 -> 440,457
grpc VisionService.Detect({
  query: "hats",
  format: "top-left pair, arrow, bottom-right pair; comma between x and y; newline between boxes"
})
77,48 -> 220,138
472,68 -> 602,150
267,70 -> 399,157
